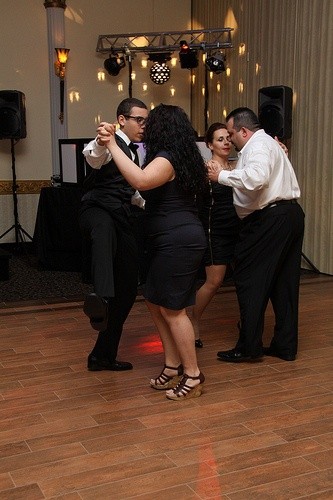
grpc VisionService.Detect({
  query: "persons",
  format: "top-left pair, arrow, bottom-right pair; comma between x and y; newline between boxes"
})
187,123 -> 288,350
96,103 -> 208,401
76,98 -> 151,370
208,107 -> 307,361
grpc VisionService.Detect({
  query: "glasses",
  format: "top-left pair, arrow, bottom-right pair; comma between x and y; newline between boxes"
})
123,114 -> 149,127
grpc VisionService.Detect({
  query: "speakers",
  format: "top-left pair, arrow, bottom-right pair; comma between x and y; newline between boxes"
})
258,85 -> 292,140
0,89 -> 26,140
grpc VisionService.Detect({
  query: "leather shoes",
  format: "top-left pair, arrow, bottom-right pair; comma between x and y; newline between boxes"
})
263,346 -> 296,361
217,349 -> 259,362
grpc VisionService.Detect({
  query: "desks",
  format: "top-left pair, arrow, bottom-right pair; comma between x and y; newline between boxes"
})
31,186 -> 84,272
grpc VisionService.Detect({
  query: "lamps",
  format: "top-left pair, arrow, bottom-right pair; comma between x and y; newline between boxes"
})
178,50 -> 200,70
149,62 -> 171,85
205,50 -> 227,74
104,55 -> 127,76
54,47 -> 71,124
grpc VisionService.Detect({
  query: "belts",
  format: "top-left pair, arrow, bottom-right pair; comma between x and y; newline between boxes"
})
269,199 -> 296,208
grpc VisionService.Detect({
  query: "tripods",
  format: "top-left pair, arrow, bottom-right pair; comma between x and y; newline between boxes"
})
0,139 -> 33,260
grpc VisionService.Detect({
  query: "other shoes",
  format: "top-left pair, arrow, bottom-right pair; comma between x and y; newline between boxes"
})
83,293 -> 110,331
87,359 -> 133,371
195,337 -> 204,348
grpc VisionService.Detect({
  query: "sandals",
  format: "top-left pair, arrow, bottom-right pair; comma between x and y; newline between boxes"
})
149,364 -> 184,389
165,371 -> 205,401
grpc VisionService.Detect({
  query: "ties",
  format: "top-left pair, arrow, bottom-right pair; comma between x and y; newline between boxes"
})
129,141 -> 139,167
